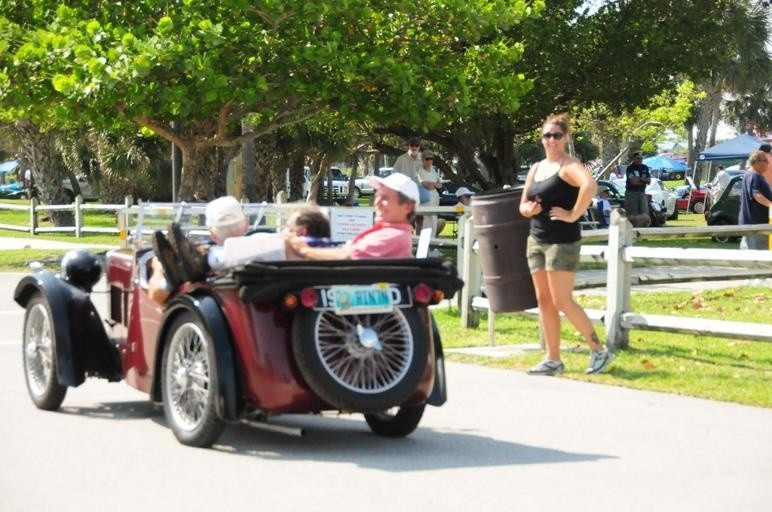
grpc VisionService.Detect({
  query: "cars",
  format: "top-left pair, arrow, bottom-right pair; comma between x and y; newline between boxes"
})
433,163 -> 687,236
11,190 -> 470,455
673,176 -> 707,214
1,182 -> 31,199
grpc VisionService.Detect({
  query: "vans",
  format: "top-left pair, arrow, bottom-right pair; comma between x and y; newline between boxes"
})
285,163 -> 350,201
59,170 -> 99,201
704,168 -> 749,244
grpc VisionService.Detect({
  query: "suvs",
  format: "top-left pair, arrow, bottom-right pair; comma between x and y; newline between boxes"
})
353,164 -> 393,197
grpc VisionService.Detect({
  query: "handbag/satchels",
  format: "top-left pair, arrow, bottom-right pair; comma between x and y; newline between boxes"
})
418,181 -> 431,206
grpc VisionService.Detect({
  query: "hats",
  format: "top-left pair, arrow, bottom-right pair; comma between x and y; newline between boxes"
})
407,136 -> 420,146
628,151 -> 646,159
367,172 -> 421,203
204,195 -> 246,228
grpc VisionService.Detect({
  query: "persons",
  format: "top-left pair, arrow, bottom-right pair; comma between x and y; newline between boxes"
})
625,153 -> 651,213
151,173 -> 419,291
599,185 -> 652,227
759,144 -> 772,187
707,165 -> 730,202
393,136 -> 423,182
149,195 -> 250,302
268,206 -> 330,249
519,113 -> 614,375
416,149 -> 442,239
454,186 -> 476,222
738,151 -> 772,249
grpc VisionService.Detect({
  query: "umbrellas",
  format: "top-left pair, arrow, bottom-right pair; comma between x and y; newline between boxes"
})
641,154 -> 686,179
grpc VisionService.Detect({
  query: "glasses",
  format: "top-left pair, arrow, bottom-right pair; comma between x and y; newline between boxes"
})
423,157 -> 434,161
541,131 -> 565,141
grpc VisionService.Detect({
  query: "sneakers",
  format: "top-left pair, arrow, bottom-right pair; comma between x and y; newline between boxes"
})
525,357 -> 565,377
167,221 -> 207,280
151,228 -> 187,288
584,348 -> 614,377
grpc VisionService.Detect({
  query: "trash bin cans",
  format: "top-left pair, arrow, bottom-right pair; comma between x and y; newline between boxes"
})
470,186 -> 538,314
